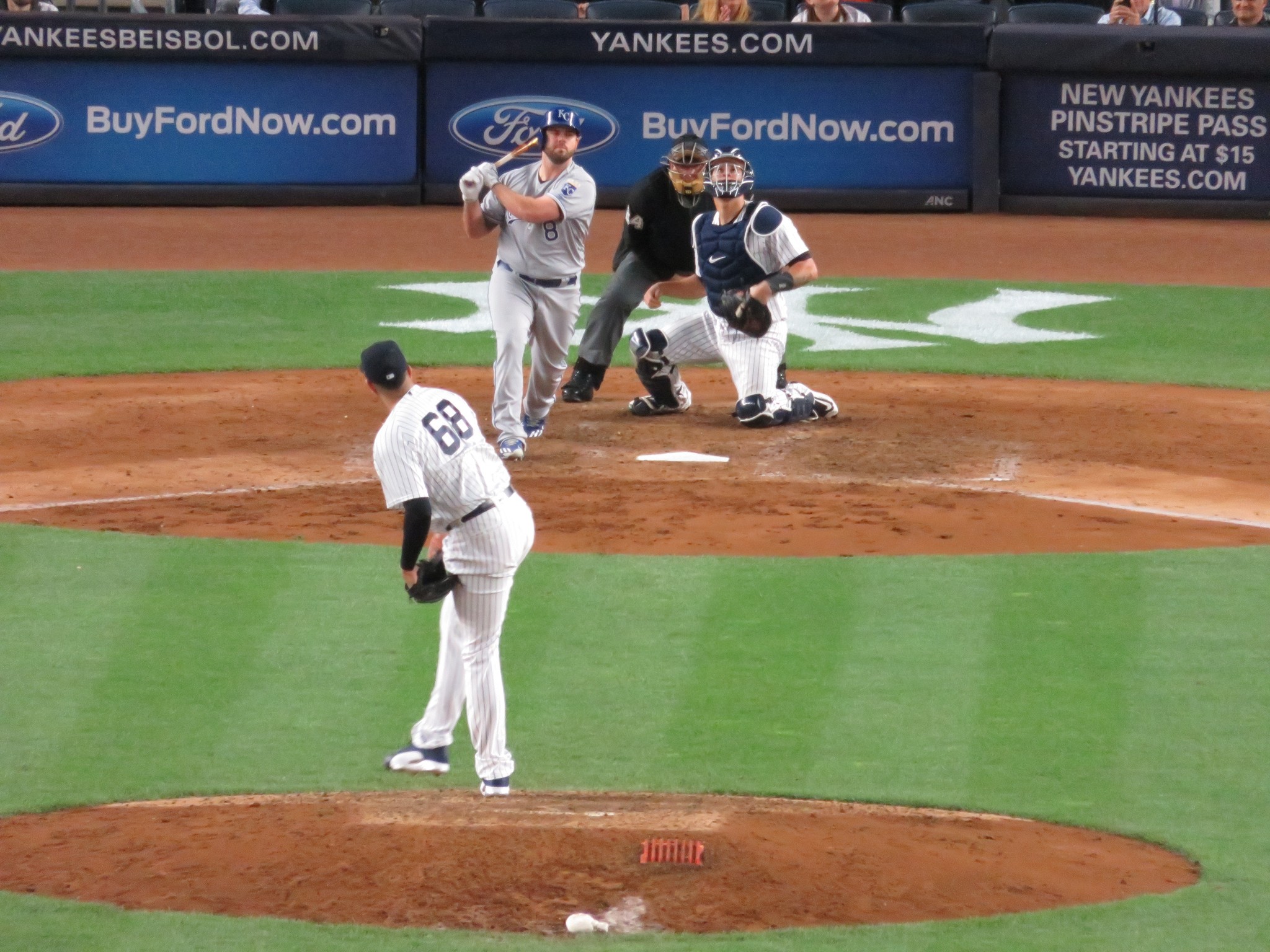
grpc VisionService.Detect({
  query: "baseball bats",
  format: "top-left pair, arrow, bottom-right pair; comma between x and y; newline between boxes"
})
462,136 -> 540,187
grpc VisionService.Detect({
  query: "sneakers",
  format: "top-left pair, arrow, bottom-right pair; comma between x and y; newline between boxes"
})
498,438 -> 523,461
788,381 -> 838,419
521,415 -> 545,436
629,393 -> 680,414
384,742 -> 451,773
481,775 -> 510,796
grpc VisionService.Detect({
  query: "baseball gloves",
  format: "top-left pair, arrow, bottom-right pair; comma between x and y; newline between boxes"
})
404,557 -> 456,603
718,283 -> 772,337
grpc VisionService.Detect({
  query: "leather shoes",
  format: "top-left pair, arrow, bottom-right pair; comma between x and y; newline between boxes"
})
562,361 -> 594,401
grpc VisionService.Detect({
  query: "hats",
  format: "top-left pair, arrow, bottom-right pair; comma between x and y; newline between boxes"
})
362,340 -> 407,381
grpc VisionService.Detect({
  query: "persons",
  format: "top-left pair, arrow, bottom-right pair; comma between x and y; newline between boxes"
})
630,146 -> 838,428
562,134 -> 786,403
0,0 -> 1270,28
360,340 -> 536,798
460,106 -> 595,462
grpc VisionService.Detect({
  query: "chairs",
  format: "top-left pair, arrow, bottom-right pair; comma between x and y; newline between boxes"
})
276,0 -> 1270,26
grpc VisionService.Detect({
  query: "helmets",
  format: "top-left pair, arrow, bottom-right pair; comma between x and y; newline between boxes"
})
540,107 -> 580,142
667,134 -> 711,195
708,147 -> 755,197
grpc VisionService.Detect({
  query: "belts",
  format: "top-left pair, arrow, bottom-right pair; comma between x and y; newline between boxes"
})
498,260 -> 576,287
445,485 -> 514,529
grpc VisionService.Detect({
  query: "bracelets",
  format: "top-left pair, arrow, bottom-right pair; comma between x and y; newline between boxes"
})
766,271 -> 793,294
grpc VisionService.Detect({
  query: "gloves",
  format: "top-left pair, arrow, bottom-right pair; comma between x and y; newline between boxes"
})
459,165 -> 484,202
479,161 -> 499,188
